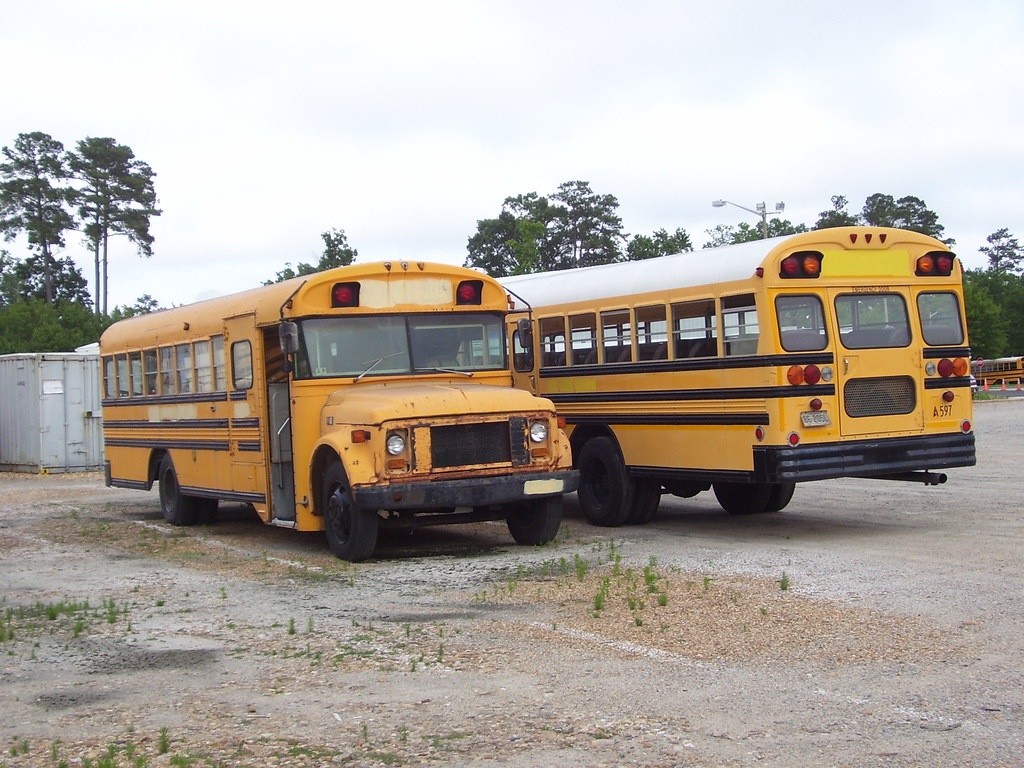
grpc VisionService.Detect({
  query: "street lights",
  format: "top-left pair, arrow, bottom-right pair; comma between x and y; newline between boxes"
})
711,198 -> 785,240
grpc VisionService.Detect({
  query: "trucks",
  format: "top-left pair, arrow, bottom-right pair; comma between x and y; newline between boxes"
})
456,227 -> 977,528
98,258 -> 583,563
971,356 -> 1024,385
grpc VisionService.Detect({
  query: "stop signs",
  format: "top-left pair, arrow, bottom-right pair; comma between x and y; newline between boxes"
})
977,357 -> 983,367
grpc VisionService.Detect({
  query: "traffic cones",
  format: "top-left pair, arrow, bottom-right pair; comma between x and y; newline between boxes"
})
982,378 -> 989,392
1000,379 -> 1007,390
1015,377 -> 1022,391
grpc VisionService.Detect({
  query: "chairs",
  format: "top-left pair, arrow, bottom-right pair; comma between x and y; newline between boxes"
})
507,325 -> 959,369
